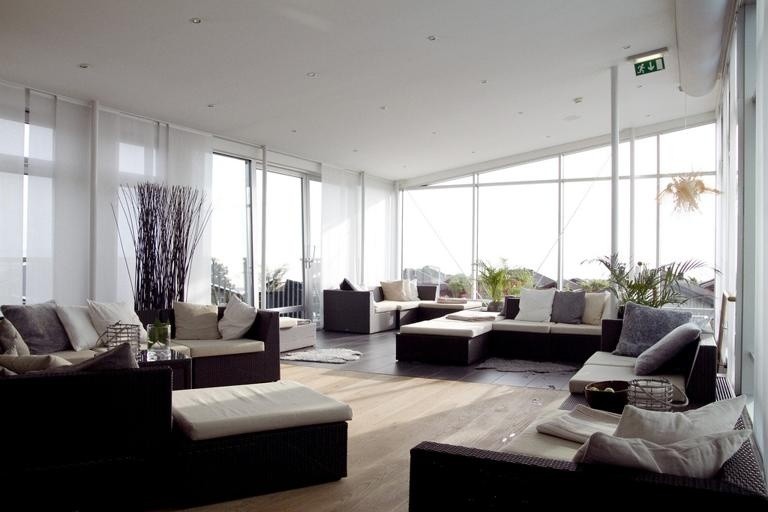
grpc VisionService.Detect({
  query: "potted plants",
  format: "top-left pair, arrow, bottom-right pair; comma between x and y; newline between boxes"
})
581,255 -> 707,318
470,258 -> 534,311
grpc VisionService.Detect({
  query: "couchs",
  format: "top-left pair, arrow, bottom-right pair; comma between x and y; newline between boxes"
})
492,296 -> 626,363
395,307 -> 506,368
0,367 -> 353,512
323,285 -> 481,335
0,306 -> 281,390
408,315 -> 768,512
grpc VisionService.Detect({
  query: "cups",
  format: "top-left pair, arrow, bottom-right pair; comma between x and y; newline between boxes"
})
147,324 -> 173,363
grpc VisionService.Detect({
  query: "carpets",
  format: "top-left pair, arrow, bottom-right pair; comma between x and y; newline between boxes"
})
280,348 -> 364,364
172,364 -> 594,512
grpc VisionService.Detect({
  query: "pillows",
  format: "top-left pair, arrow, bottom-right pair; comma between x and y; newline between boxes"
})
403,280 -> 414,301
613,394 -> 748,444
0,355 -> 73,374
572,430 -> 755,479
634,322 -> 702,376
611,301 -> 692,357
513,287 -> 557,323
0,317 -> 30,356
379,280 -> 408,301
51,303 -> 105,352
86,299 -> 153,344
410,279 -> 421,301
0,300 -> 69,355
339,278 -> 358,291
172,300 -> 221,340
217,294 -> 257,340
582,293 -> 610,326
550,290 -> 586,325
26,342 -> 140,374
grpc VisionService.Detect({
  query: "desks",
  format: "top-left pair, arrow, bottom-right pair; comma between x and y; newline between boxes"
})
279,316 -> 317,353
94,349 -> 192,390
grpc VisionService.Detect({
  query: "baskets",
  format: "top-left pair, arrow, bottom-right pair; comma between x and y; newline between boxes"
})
95,320 -> 143,363
626,377 -> 691,414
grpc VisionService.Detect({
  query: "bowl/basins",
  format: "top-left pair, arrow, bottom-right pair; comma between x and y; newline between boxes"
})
583,378 -> 633,415
